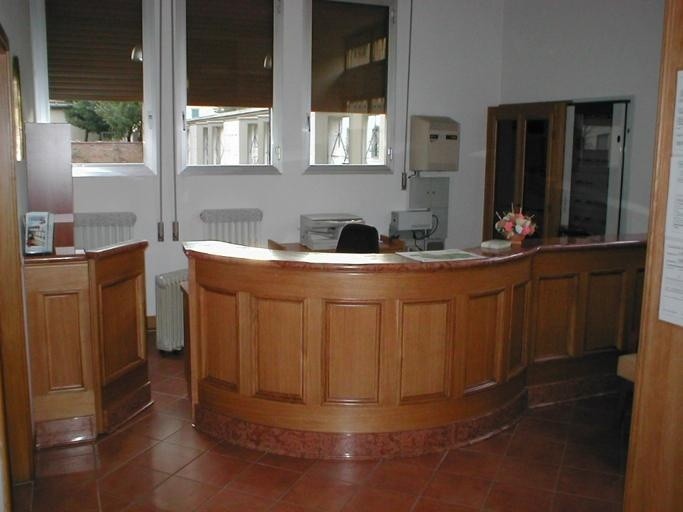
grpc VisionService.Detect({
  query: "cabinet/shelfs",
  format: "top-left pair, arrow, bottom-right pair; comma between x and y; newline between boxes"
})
481,101 -> 566,243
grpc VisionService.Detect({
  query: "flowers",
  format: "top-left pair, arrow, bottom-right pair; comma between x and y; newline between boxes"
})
494,202 -> 538,240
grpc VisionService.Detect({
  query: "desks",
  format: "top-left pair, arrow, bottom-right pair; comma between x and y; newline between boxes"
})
268,234 -> 407,255
182,241 -> 538,459
526,234 -> 647,410
22,240 -> 158,451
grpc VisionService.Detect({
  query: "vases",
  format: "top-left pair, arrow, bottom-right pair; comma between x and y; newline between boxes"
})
508,234 -> 525,246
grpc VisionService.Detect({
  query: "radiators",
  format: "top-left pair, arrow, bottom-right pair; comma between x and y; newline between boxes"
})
75,211 -> 138,252
154,270 -> 189,354
199,209 -> 264,248
